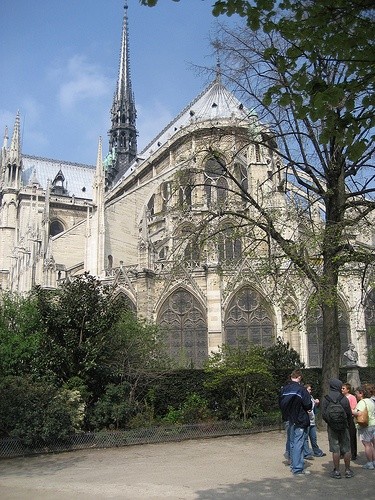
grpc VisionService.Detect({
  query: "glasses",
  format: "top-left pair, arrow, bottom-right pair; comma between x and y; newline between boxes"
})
341,388 -> 347,390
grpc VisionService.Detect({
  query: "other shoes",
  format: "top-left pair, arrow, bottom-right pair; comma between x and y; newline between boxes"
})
362,462 -> 375,470
285,452 -> 287,459
351,455 -> 357,460
340,454 -> 344,459
343,471 -> 352,478
330,471 -> 341,479
305,456 -> 314,460
293,470 -> 311,477
317,453 -> 326,457
288,457 -> 291,465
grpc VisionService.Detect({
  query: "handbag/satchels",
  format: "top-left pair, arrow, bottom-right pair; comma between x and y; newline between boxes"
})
297,385 -> 310,429
313,405 -> 319,414
356,399 -> 368,428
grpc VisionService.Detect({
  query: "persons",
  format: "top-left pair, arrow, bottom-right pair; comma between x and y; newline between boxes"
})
278,368 -> 326,474
322,377 -> 356,479
340,383 -> 357,460
351,384 -> 375,470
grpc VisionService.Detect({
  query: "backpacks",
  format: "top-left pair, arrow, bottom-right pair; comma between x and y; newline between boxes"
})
324,394 -> 347,432
